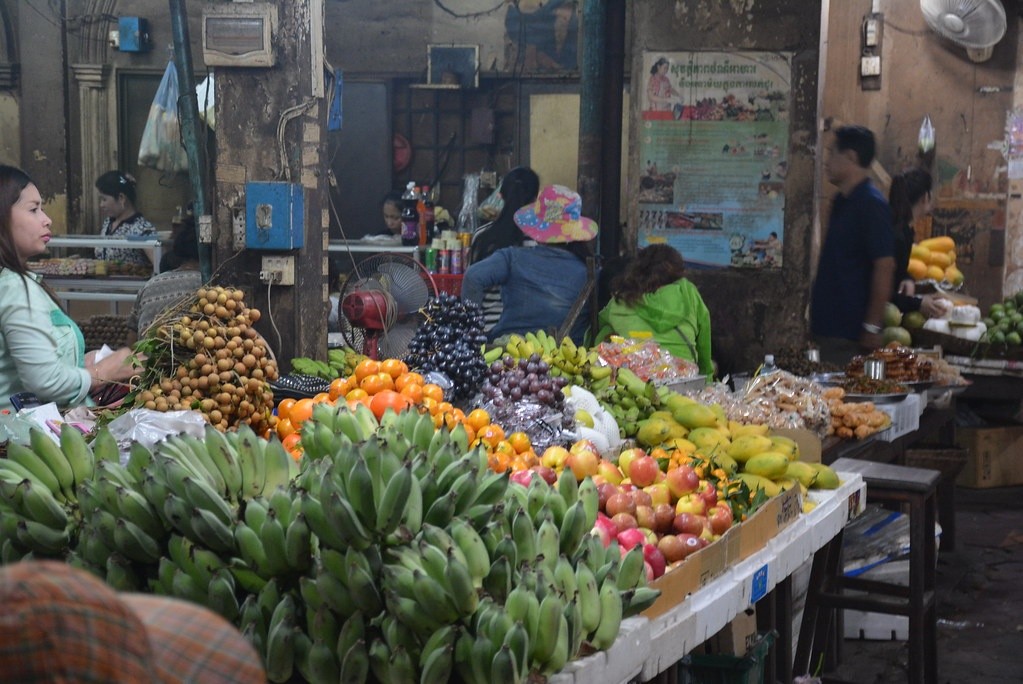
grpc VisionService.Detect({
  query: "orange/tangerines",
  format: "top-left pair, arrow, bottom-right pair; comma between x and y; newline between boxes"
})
265,358 -> 537,480
649,446 -> 757,522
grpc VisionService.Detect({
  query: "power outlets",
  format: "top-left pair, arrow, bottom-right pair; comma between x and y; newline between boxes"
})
231,207 -> 247,252
109,31 -> 119,47
261,256 -> 295,285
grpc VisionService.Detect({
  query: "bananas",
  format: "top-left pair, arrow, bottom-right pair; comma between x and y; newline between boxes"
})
481,329 -> 675,438
1,402 -> 664,683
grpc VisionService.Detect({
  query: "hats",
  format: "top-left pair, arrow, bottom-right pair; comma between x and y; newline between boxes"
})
513,185 -> 598,243
0,562 -> 265,684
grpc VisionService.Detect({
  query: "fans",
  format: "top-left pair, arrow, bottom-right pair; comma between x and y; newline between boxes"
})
920,0 -> 1006,63
337,251 -> 439,361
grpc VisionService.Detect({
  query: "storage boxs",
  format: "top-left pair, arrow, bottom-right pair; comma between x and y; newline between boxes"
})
843,553 -> 939,640
874,393 -> 922,443
952,421 -> 1023,489
684,599 -> 776,683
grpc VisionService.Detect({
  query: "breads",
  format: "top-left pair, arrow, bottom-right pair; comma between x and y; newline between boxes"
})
872,340 -> 966,387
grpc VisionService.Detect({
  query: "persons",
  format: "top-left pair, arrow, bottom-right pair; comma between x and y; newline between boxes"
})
468,167 -> 541,332
0,164 -> 146,413
127,216 -> 202,345
647,58 -> 684,111
95,171 -> 157,270
811,126 -> 898,364
889,171 -> 932,302
462,186 -> 599,346
583,244 -> 713,382
377,194 -> 405,235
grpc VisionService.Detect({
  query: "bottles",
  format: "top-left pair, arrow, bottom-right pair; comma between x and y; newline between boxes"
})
759,354 -> 778,375
400,182 -> 434,247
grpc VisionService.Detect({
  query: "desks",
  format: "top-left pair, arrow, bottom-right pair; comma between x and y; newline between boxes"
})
827,379 -> 978,555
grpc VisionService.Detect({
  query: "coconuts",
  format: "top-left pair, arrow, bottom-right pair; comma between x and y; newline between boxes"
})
884,303 -> 924,345
985,289 -> 1023,346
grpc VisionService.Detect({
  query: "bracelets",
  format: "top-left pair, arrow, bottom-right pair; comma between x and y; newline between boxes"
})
862,322 -> 881,333
94,366 -> 100,385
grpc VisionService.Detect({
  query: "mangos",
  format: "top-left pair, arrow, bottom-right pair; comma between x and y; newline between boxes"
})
630,393 -> 845,497
801,498 -> 816,512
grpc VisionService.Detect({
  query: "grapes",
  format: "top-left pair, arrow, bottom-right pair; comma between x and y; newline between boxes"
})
398,288 -> 572,455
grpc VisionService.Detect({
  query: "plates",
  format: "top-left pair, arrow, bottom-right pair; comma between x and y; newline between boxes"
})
813,370 -> 935,405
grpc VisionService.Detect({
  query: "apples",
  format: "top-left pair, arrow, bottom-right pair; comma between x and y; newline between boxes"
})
511,440 -> 734,586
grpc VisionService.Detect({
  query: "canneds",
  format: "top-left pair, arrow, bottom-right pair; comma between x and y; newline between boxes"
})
425,231 -> 472,275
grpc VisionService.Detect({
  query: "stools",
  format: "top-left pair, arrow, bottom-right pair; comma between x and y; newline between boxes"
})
794,457 -> 942,684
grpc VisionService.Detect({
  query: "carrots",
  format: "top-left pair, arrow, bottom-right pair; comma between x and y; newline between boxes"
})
699,372 -> 886,439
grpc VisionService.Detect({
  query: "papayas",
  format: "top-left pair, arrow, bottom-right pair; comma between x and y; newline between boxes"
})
908,235 -> 962,286
